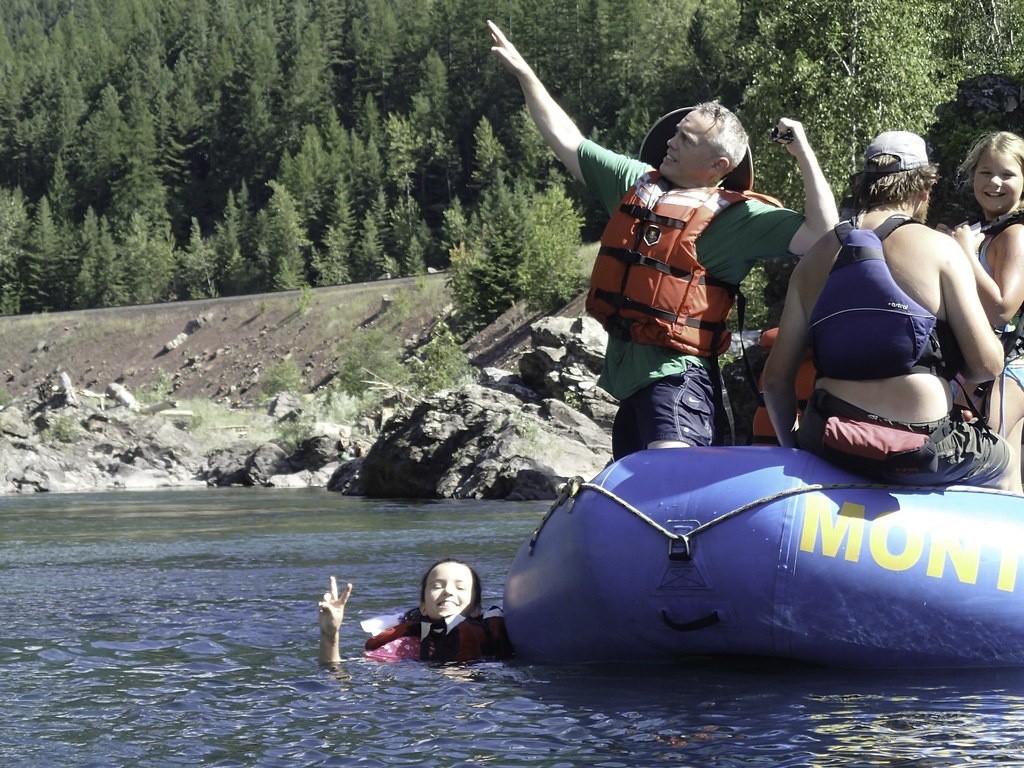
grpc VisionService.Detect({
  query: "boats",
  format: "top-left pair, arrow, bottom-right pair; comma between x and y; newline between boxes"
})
503,447 -> 1023,678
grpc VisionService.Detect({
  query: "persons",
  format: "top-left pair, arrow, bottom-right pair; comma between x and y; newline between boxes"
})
761,131 -> 1024,496
485,18 -> 840,459
318,558 -> 504,664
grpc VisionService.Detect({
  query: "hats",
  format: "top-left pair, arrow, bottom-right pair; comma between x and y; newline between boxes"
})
864,130 -> 929,172
